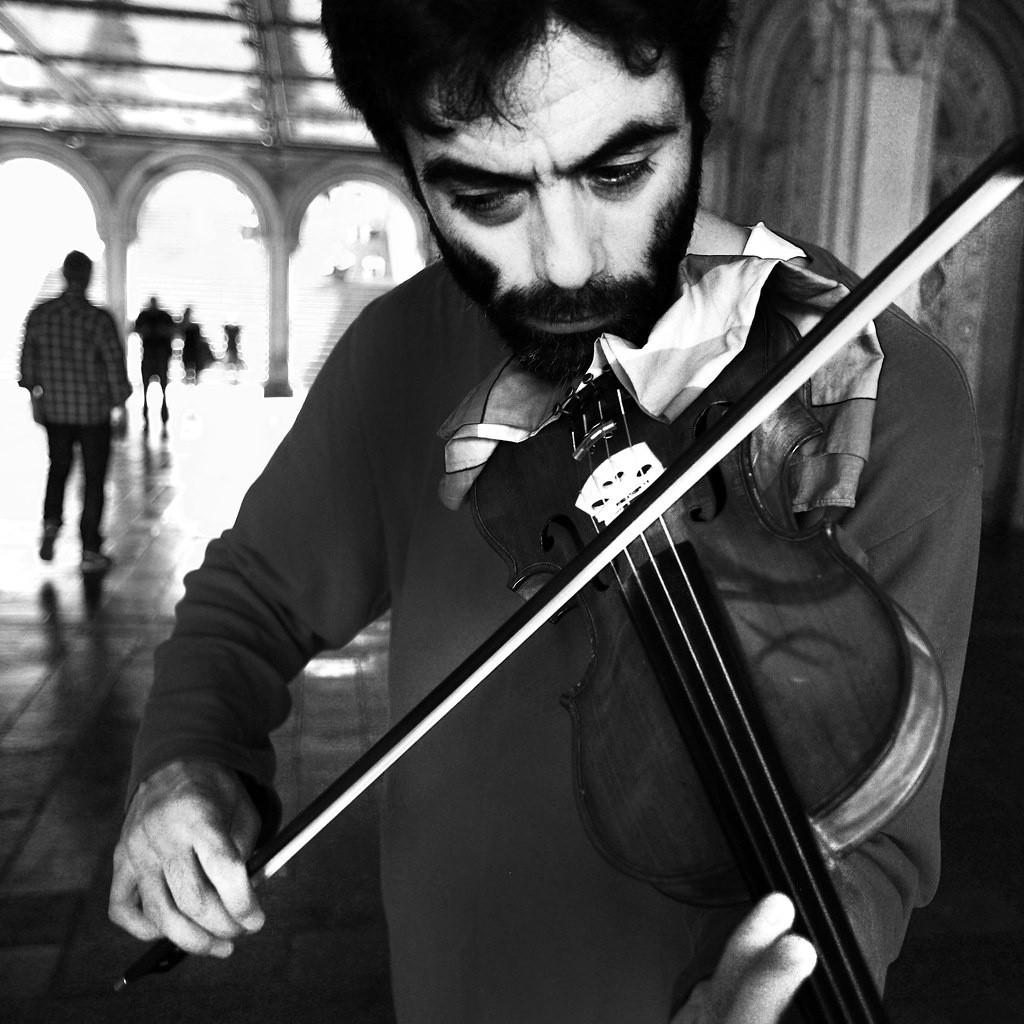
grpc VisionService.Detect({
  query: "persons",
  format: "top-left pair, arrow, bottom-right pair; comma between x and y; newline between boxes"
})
108,0 -> 982,1024
135,298 -> 175,424
178,307 -> 215,385
222,321 -> 246,381
18,250 -> 133,574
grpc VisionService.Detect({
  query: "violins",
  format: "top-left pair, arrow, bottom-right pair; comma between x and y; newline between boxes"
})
466,297 -> 955,1024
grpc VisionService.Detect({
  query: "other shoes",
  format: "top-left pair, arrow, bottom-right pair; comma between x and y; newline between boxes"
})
81,550 -> 111,574
40,524 -> 57,560
